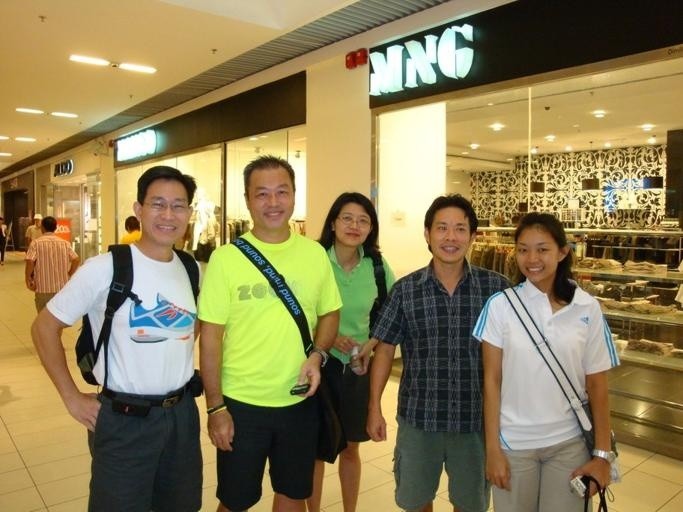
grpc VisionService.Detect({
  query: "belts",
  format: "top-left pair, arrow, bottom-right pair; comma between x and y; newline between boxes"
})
103,381 -> 191,408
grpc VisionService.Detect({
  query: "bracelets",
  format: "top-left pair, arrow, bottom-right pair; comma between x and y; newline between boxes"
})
206,404 -> 227,414
309,348 -> 329,366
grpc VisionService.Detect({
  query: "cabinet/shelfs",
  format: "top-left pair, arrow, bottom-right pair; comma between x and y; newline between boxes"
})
574,266 -> 682,383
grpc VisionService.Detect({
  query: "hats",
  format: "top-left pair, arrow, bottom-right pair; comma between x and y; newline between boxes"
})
34,214 -> 43,220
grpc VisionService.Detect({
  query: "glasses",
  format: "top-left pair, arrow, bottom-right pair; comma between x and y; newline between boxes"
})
336,214 -> 371,226
141,198 -> 191,212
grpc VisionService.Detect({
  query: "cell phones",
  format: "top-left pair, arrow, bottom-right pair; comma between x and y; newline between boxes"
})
289,384 -> 312,396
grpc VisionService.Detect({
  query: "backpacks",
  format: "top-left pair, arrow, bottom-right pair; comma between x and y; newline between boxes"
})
76,244 -> 200,387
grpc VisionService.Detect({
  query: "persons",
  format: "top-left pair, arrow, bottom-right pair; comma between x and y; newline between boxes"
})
23,215 -> 81,347
0,216 -> 7,262
119,215 -> 142,244
24,212 -> 45,249
306,194 -> 398,512
472,213 -> 621,512
198,152 -> 341,512
30,165 -> 205,512
367,195 -> 511,512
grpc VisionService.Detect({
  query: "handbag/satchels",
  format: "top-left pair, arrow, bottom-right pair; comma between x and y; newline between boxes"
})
574,398 -> 618,461
309,378 -> 348,465
367,250 -> 389,353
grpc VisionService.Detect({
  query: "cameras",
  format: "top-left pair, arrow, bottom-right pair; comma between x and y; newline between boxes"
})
568,474 -> 587,499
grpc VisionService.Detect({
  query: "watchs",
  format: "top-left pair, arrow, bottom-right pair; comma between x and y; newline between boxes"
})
592,447 -> 617,462
